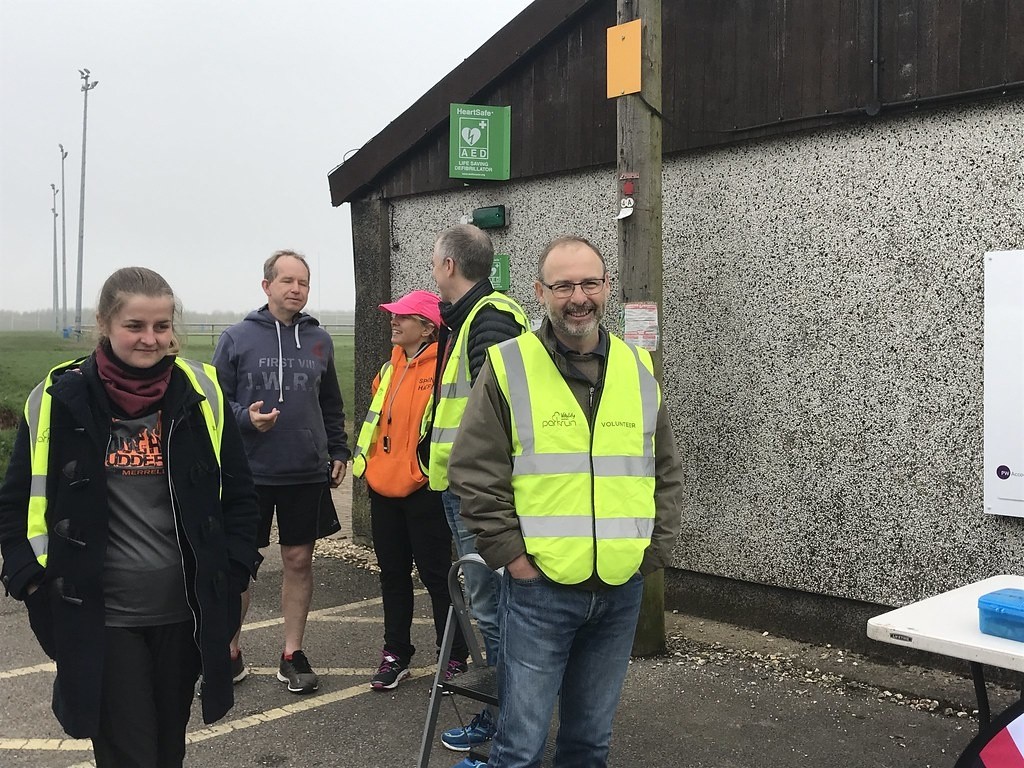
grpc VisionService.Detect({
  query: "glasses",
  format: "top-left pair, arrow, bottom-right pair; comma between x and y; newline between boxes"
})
391,313 -> 429,324
543,279 -> 605,299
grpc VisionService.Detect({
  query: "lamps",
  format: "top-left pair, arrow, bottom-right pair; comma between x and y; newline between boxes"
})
471,203 -> 512,230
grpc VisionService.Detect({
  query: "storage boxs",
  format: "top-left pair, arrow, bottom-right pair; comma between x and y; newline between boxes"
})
978,588 -> 1024,641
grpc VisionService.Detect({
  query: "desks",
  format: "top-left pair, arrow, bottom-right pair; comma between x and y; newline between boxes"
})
867,574 -> 1024,768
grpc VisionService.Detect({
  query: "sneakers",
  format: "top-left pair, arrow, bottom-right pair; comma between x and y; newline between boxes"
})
370,650 -> 411,689
197,650 -> 247,694
450,757 -> 488,768
441,709 -> 497,752
276,650 -> 319,692
429,660 -> 468,695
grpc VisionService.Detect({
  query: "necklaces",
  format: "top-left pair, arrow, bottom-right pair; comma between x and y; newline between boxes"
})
383,344 -> 430,453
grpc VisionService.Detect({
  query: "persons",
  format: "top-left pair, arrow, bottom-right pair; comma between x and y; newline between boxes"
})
0,267 -> 259,768
197,252 -> 352,696
448,235 -> 684,767
353,291 -> 470,696
419,224 -> 530,767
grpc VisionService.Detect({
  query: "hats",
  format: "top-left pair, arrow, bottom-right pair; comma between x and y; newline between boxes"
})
378,290 -> 442,330
